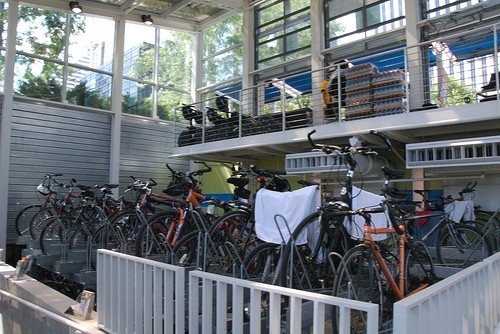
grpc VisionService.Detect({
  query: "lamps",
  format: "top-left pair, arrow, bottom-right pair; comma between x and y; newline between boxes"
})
69,1 -> 84,13
142,15 -> 153,25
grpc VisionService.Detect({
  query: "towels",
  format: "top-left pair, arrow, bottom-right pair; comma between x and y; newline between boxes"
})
344,186 -> 392,240
444,200 -> 477,224
253,183 -> 326,264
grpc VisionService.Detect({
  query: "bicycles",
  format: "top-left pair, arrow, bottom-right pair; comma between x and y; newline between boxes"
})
16,129 -> 500,334
